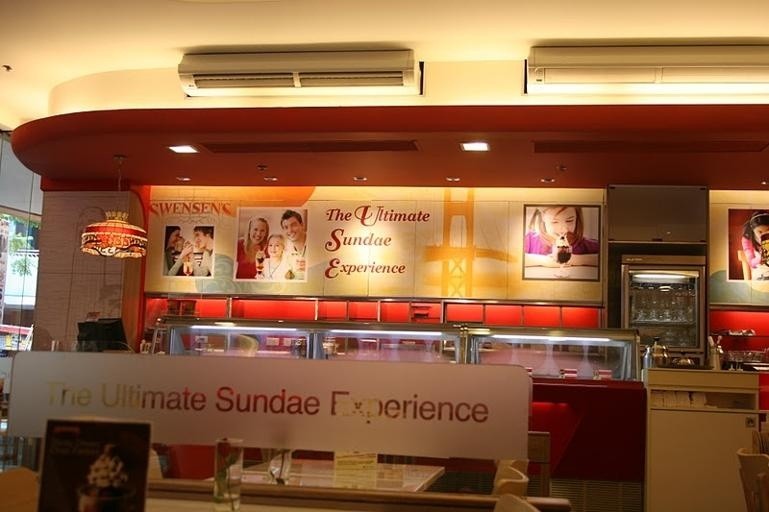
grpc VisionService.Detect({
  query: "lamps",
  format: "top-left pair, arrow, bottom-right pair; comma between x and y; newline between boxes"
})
72,151 -> 148,261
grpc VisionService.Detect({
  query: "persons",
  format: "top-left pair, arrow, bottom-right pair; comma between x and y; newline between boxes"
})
163,225 -> 213,277
524,204 -> 599,268
237,210 -> 305,282
741,211 -> 769,280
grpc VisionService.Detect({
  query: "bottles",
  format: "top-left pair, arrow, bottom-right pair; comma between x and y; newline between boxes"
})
663,326 -> 692,346
652,336 -> 669,367
704,346 -> 721,370
641,346 -> 655,368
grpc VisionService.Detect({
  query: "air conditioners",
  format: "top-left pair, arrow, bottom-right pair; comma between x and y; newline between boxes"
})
524,41 -> 764,94
176,45 -> 424,97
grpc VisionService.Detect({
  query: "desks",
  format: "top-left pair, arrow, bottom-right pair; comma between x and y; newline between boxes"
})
204,457 -> 446,492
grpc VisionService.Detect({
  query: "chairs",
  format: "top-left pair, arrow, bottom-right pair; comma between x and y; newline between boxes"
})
491,459 -> 538,510
734,424 -> 768,512
148,452 -> 162,479
1,466 -> 39,510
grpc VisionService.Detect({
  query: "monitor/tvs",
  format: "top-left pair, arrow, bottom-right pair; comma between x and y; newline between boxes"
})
78,318 -> 128,353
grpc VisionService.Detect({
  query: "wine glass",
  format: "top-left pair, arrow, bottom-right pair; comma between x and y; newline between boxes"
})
550,245 -> 573,278
631,293 -> 689,323
728,350 -> 763,372
256,255 -> 266,279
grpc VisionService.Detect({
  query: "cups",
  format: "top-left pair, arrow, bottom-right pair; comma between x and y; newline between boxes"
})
139,342 -> 151,355
291,250 -> 302,273
50,340 -> 77,351
